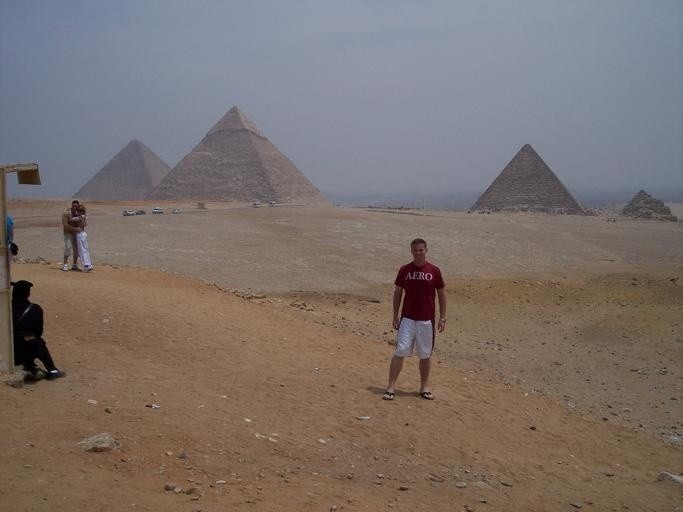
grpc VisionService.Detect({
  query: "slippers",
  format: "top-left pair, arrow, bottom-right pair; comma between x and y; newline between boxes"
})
383,390 -> 395,400
419,392 -> 434,400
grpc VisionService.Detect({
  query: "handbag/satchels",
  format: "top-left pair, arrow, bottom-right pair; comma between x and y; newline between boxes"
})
10,243 -> 18,255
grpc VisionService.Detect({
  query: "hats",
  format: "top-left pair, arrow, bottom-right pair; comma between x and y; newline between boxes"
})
10,280 -> 33,287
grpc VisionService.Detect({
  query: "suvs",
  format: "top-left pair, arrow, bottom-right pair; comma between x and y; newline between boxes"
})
123,206 -> 181,216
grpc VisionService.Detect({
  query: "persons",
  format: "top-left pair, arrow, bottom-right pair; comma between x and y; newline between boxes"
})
62,200 -> 86,272
7,212 -> 16,282
67,204 -> 94,273
12,279 -> 67,383
382,237 -> 447,402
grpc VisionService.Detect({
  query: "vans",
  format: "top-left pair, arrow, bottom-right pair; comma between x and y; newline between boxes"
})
253,201 -> 277,208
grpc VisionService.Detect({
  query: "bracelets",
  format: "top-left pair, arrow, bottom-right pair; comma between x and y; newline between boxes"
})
439,318 -> 447,323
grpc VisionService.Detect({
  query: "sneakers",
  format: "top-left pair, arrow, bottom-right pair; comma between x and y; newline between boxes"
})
84,264 -> 94,273
71,264 -> 83,271
32,367 -> 65,381
62,264 -> 68,270
